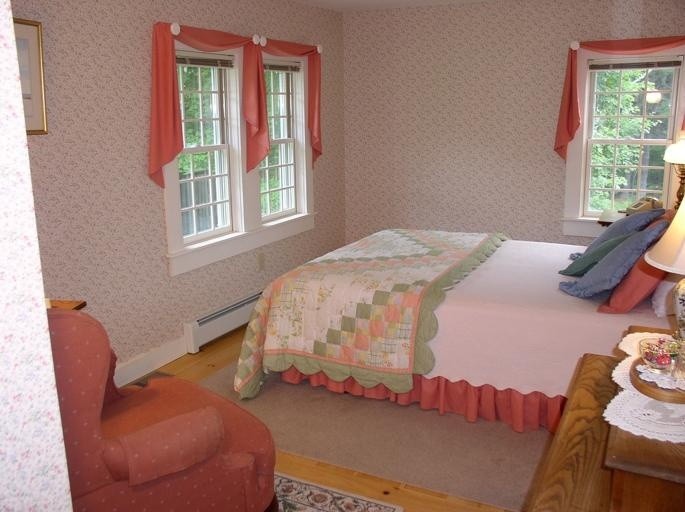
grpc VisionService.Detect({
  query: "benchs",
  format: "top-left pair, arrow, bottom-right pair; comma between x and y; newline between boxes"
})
528,352 -> 619,512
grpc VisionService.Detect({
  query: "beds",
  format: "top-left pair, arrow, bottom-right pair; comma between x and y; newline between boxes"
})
257,226 -> 685,432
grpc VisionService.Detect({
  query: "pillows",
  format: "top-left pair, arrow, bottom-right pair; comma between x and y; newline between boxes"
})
557,207 -> 677,314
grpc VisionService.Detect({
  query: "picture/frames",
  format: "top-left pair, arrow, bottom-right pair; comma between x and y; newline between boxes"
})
12,17 -> 48,136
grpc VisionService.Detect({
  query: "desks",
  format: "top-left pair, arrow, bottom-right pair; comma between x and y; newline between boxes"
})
602,322 -> 685,511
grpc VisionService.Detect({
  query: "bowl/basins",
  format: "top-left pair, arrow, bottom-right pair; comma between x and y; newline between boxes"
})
638,337 -> 679,367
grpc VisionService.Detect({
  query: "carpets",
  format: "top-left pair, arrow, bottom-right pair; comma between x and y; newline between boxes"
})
199,358 -> 547,512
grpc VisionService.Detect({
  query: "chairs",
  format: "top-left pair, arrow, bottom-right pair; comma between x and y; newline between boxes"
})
46,307 -> 285,511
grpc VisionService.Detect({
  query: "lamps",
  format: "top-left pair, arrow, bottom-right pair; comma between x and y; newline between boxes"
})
664,128 -> 685,210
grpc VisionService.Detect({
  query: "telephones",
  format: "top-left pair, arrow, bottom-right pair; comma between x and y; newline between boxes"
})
627,196 -> 663,215
